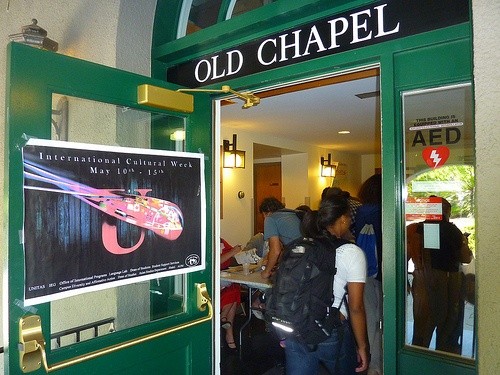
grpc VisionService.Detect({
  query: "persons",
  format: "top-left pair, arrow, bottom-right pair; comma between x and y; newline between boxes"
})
407,195 -> 475,356
242,172 -> 382,375
220,236 -> 242,353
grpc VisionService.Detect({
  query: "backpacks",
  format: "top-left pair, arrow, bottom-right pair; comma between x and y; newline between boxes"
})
263,236 -> 358,345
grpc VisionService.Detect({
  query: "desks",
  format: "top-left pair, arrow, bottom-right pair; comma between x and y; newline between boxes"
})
221,265 -> 274,346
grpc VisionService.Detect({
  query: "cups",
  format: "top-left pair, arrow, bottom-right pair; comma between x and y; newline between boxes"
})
242,262 -> 250,275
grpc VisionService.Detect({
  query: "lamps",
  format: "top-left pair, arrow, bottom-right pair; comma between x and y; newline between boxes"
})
223,134 -> 245,168
169,128 -> 186,141
321,154 -> 336,178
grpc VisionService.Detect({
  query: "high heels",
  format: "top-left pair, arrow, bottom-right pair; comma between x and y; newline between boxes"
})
221,316 -> 229,338
224,332 -> 236,350
